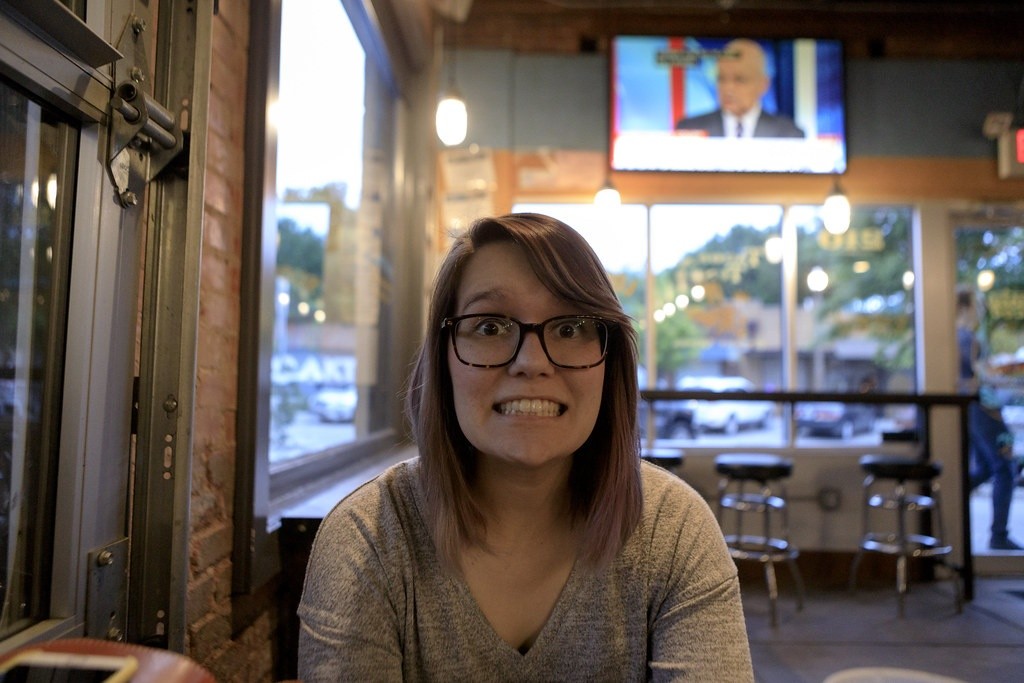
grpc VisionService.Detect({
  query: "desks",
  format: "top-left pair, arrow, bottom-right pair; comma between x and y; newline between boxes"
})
639,383 -> 981,601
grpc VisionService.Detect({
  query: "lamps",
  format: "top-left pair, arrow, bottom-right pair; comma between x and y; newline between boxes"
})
435,0 -> 467,146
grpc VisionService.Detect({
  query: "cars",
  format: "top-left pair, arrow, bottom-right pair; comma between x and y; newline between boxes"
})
679,378 -> 777,436
312,386 -> 358,424
638,384 -> 701,439
966,391 -> 1024,487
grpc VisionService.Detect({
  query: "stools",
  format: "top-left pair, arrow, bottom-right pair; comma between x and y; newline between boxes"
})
710,452 -> 805,629
851,453 -> 963,618
637,447 -> 684,476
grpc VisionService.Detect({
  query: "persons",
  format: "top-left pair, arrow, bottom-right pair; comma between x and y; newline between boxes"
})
295,211 -> 759,683
665,38 -> 809,138
951,282 -> 1024,551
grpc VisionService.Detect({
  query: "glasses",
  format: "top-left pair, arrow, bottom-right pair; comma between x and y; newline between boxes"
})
439,314 -> 609,370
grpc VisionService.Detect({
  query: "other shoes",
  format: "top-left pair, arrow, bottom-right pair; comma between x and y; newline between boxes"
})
989,530 -> 1021,548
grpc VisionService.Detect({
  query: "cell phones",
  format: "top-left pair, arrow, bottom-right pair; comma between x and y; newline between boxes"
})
608,32 -> 849,174
0,650 -> 139,683
